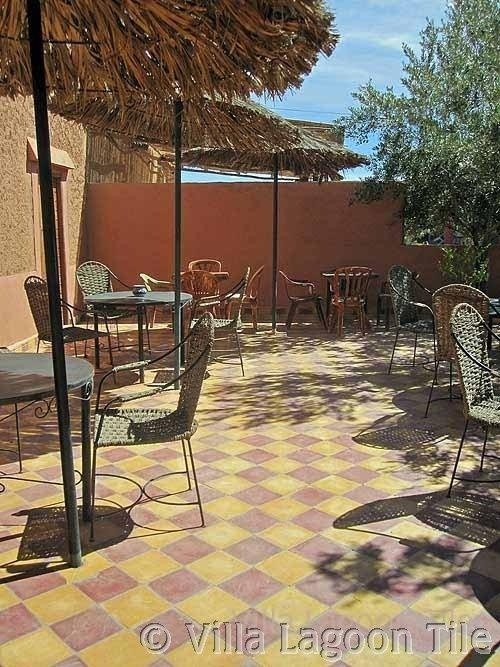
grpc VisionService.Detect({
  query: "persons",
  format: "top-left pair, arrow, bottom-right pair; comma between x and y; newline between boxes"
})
429,216 -> 466,245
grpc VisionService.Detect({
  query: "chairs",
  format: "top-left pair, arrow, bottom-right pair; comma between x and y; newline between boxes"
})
278,264 -> 500,499
1,259 -> 265,542
